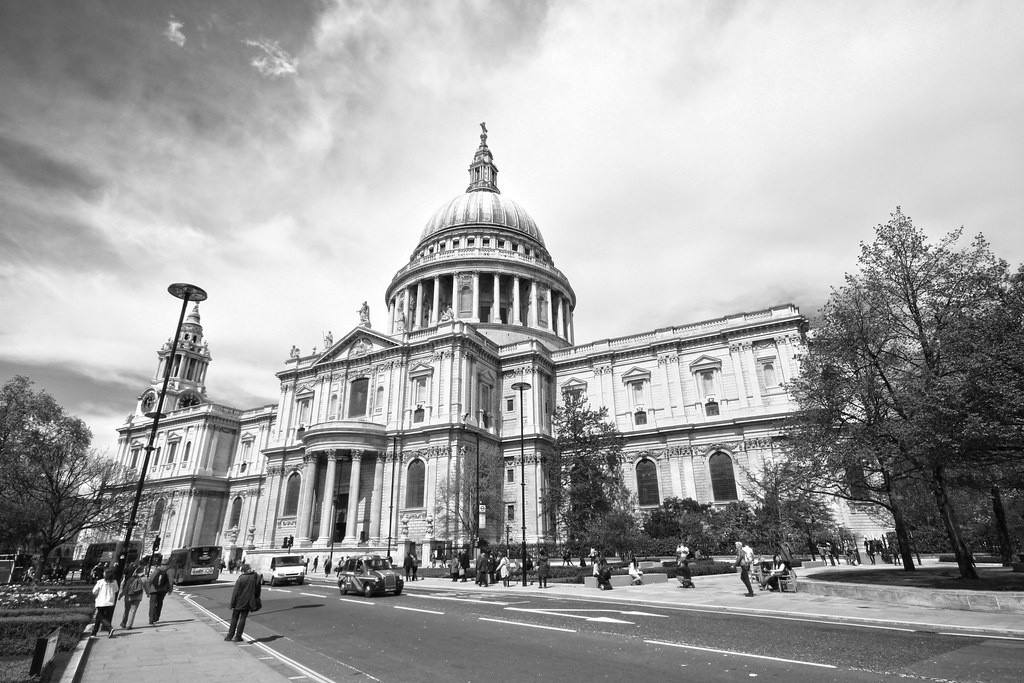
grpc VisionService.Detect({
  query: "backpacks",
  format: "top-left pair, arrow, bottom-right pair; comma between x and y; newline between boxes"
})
743,545 -> 754,563
153,567 -> 170,587
127,574 -> 143,596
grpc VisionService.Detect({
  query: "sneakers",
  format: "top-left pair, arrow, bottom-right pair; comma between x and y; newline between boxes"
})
126,626 -> 132,630
108,628 -> 115,638
119,623 -> 126,628
224,636 -> 232,641
234,636 -> 243,642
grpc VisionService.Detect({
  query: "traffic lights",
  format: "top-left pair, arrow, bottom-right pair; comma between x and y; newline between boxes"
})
154,536 -> 161,551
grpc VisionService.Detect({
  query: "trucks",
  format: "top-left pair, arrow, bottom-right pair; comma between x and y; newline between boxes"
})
244,552 -> 306,587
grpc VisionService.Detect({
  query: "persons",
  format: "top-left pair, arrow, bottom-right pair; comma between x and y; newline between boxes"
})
25,558 -> 185,599
117,566 -> 145,631
145,564 -> 175,624
223,564 -> 262,642
86,567 -> 119,640
211,531 -> 921,600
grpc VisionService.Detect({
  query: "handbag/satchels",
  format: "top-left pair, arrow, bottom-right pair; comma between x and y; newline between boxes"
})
506,562 -> 510,571
458,566 -> 465,576
249,596 -> 262,613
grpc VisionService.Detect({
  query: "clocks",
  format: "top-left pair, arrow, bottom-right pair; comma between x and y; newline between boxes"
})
179,393 -> 202,408
141,392 -> 156,415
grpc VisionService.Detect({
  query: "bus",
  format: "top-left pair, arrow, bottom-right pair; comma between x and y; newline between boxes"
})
78,539 -> 142,581
168,545 -> 222,585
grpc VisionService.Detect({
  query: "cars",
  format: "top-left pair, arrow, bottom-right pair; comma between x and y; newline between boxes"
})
336,554 -> 404,597
